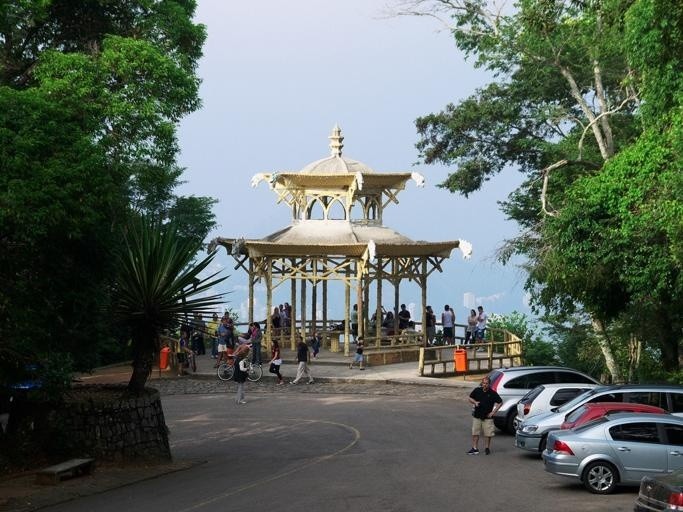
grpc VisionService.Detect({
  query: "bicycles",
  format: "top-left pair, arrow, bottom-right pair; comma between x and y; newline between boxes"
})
217,354 -> 264,383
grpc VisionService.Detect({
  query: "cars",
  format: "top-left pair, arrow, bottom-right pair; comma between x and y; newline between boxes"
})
539,411 -> 682,494
513,382 -> 606,429
0,363 -> 47,394
560,401 -> 674,432
631,465 -> 682,512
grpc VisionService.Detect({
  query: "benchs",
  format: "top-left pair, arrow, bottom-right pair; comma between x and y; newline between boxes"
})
36,458 -> 97,485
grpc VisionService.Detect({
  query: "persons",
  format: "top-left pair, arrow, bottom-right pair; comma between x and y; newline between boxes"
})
195,313 -> 206,356
425,306 -> 436,347
234,321 -> 262,383
177,330 -> 195,377
289,336 -> 316,384
350,337 -> 365,370
371,306 -> 388,321
476,306 -> 486,352
351,304 -> 359,344
399,304 -> 410,335
465,376 -> 504,455
463,309 -> 478,349
311,332 -> 323,360
268,340 -> 284,384
209,312 -> 235,368
270,302 -> 292,337
383,312 -> 395,336
442,305 -> 455,346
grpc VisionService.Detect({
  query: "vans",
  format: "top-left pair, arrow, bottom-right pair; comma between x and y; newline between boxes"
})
513,382 -> 682,451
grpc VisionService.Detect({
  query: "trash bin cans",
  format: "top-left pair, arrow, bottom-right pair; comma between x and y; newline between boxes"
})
159,347 -> 172,369
453,349 -> 468,373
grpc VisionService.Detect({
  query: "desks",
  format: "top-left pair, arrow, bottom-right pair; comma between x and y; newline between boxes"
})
316,330 -> 352,352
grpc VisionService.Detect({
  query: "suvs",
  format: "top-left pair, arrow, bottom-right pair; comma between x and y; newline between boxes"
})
482,363 -> 606,437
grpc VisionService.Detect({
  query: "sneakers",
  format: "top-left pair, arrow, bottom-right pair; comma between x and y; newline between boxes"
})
467,448 -> 480,455
485,448 -> 490,455
